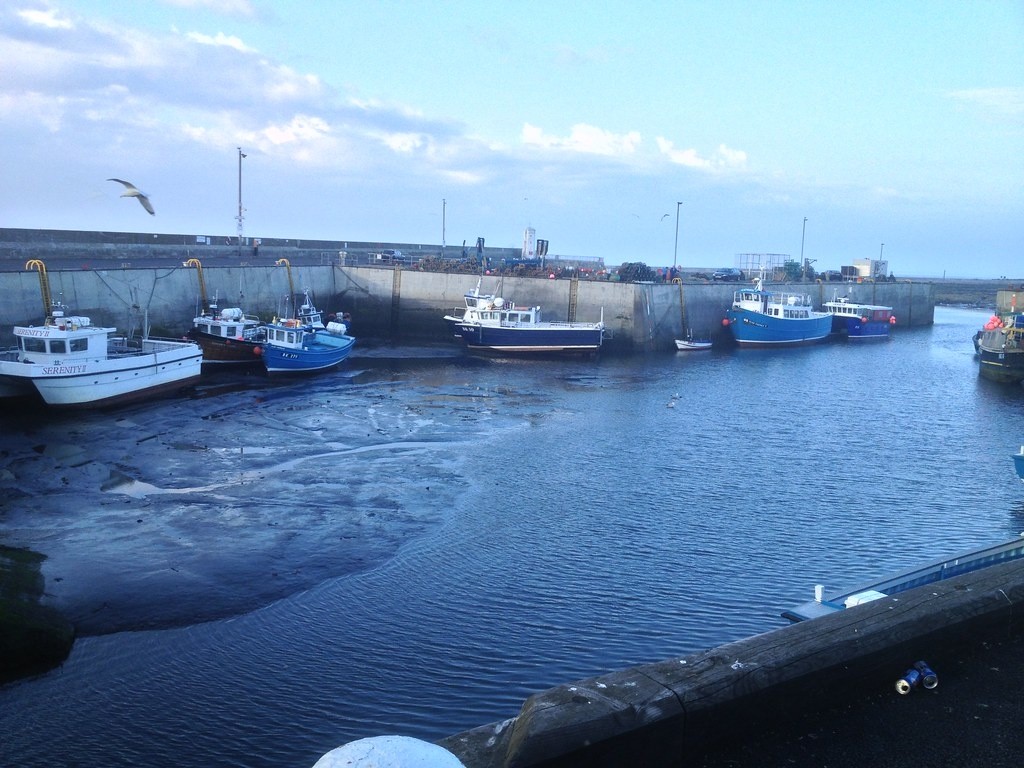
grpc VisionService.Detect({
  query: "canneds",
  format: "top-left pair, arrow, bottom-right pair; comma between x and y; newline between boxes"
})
913,660 -> 938,689
895,670 -> 920,694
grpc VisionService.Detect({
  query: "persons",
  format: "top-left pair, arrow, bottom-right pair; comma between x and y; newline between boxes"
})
657,266 -> 679,283
342,313 -> 352,335
322,314 -> 336,328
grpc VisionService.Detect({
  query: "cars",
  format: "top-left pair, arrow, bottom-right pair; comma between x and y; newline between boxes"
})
381,249 -> 406,262
713,268 -> 741,281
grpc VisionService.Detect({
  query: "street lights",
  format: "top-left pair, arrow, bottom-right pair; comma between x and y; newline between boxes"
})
880,243 -> 885,260
800,217 -> 808,265
674,201 -> 683,267
237,147 -> 248,253
443,198 -> 446,248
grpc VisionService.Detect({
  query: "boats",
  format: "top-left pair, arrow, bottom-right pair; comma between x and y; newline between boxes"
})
726,264 -> 832,343
674,328 -> 713,350
973,288 -> 1024,383
262,289 -> 356,372
821,288 -> 896,337
456,277 -> 606,351
443,278 -> 494,337
0,291 -> 204,404
185,287 -> 267,363
